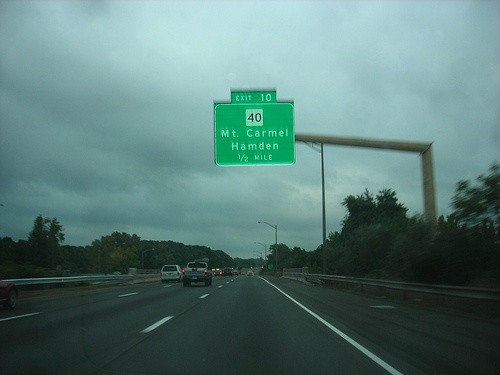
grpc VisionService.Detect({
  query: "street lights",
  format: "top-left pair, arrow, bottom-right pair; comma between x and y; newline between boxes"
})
252,241 -> 267,269
257,220 -> 278,272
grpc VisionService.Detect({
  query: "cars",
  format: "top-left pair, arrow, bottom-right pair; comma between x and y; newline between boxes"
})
214,268 -> 238,276
241,267 -> 255,277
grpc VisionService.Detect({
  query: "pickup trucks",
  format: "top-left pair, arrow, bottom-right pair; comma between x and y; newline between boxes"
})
183,261 -> 212,286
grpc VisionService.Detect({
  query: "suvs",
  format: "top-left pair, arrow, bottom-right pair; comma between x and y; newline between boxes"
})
161,265 -> 183,283
